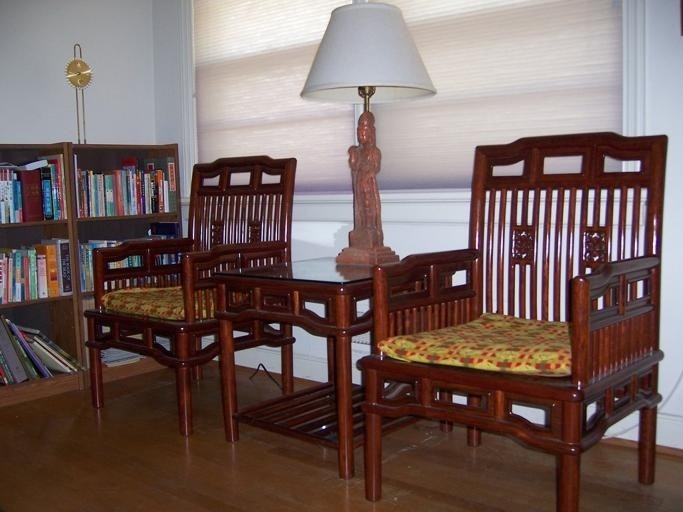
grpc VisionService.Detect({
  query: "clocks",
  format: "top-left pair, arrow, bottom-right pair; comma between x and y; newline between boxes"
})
64,56 -> 94,90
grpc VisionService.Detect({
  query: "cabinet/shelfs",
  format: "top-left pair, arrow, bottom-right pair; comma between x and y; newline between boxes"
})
0,144 -> 86,406
71,140 -> 186,388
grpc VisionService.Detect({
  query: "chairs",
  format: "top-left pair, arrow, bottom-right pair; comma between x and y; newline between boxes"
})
90,155 -> 298,437
355,128 -> 670,510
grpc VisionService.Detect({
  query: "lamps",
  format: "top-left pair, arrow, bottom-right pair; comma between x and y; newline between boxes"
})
300,2 -> 442,274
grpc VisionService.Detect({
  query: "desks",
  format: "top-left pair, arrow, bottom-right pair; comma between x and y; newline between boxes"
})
213,246 -> 372,482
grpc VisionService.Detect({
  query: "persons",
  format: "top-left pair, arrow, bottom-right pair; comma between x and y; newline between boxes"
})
335,112 -> 400,268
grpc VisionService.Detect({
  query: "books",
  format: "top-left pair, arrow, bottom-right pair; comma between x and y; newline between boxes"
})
1,317 -> 87,387
99,326 -> 141,368
73,153 -> 177,218
1,238 -> 73,305
77,223 -> 184,293
1,151 -> 67,225
